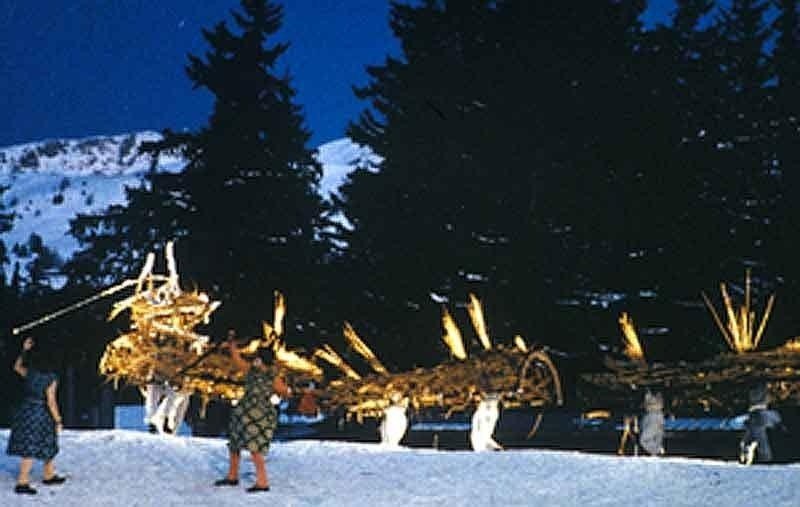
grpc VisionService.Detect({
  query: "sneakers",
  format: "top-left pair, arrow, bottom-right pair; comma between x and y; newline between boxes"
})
15,484 -> 36,494
247,485 -> 270,491
42,474 -> 66,485
216,478 -> 238,487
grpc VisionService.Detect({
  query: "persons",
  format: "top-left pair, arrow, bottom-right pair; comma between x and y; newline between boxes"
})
210,340 -> 289,497
3,323 -> 70,496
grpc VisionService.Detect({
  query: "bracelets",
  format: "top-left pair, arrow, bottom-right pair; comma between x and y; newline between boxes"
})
55,420 -> 63,426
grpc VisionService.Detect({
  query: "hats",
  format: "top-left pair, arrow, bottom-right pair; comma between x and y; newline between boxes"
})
256,347 -> 273,364
